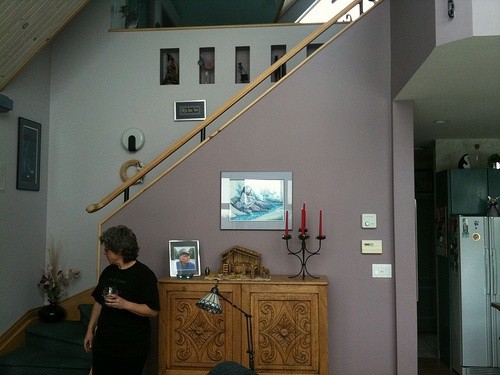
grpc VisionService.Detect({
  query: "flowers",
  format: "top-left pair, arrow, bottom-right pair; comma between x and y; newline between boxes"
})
37,237 -> 81,303
198,52 -> 213,70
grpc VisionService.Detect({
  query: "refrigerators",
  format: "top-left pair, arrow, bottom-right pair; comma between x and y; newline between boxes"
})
451,216 -> 500,375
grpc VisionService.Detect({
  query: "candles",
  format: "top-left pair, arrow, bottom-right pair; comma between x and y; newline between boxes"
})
319,209 -> 323,237
285,210 -> 289,236
301,203 -> 306,235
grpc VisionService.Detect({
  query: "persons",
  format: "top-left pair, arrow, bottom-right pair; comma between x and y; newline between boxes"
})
177,251 -> 195,270
83,225 -> 160,375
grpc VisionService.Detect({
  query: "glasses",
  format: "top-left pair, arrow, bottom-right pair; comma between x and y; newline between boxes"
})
104,248 -> 110,253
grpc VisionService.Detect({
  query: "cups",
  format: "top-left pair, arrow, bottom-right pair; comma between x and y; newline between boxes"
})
102,287 -> 118,299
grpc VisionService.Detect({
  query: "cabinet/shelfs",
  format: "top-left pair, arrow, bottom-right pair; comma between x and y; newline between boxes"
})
157,274 -> 329,375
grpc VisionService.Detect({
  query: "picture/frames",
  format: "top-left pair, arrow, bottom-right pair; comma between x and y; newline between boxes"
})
219,169 -> 293,232
15,116 -> 42,193
168,239 -> 202,277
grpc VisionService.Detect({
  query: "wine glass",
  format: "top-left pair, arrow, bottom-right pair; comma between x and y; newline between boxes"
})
202,68 -> 210,84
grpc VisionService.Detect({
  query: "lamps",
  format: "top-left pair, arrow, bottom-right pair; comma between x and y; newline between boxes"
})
195,280 -> 255,372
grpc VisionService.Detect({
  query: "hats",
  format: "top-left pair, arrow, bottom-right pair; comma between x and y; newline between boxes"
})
179,248 -> 189,255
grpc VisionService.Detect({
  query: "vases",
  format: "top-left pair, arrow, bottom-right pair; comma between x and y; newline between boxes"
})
203,70 -> 210,84
38,302 -> 69,325
